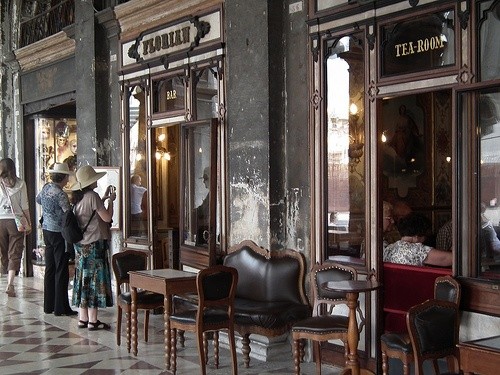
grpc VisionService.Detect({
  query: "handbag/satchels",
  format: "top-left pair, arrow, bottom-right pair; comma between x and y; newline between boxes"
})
13,213 -> 32,231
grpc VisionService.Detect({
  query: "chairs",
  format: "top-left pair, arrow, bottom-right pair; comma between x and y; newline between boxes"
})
112,249 -> 163,352
407,298 -> 464,375
169,266 -> 239,375
290,259 -> 357,375
381,274 -> 460,375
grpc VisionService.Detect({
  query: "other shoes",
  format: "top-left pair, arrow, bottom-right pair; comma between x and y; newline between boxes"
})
6,284 -> 15,297
55,308 -> 78,316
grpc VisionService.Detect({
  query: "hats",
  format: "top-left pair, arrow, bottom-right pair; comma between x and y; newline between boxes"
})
70,165 -> 107,191
48,163 -> 74,175
197,167 -> 210,179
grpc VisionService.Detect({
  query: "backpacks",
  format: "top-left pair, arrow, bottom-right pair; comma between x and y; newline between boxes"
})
61,187 -> 96,244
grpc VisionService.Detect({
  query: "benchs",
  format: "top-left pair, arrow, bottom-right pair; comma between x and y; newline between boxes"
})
383,262 -> 500,335
173,240 -> 312,369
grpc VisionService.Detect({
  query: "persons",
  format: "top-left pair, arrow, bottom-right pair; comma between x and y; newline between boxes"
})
192,166 -> 210,247
0,157 -> 32,296
36,162 -> 79,317
63,165 -> 116,331
357,193 -> 500,268
130,159 -> 147,238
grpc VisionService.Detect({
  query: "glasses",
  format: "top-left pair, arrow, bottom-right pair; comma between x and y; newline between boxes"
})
384,215 -> 393,221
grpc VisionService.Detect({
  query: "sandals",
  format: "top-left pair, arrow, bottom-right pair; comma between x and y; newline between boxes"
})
87,319 -> 111,330
78,319 -> 89,328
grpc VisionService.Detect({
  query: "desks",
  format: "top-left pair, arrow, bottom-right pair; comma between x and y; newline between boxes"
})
127,268 -> 219,371
455,335 -> 500,375
321,280 -> 381,375
